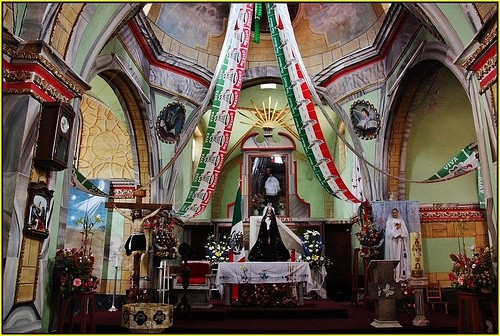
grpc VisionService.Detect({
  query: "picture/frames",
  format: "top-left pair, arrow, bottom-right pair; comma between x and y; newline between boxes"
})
247,155 -> 289,218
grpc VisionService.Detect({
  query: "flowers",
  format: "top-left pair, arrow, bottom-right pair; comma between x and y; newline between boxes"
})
48,214 -> 100,300
355,229 -> 384,245
204,231 -> 244,264
447,246 -> 496,290
300,230 -> 333,268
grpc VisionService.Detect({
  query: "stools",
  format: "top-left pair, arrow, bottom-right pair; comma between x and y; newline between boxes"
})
458,292 -> 499,334
58,292 -> 96,334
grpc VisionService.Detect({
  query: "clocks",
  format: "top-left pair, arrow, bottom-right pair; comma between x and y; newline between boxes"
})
34,98 -> 76,171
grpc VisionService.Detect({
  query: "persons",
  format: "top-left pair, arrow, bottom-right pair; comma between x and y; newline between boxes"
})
261,168 -> 279,211
112,204 -> 164,277
384,208 -> 412,282
258,207 -> 277,246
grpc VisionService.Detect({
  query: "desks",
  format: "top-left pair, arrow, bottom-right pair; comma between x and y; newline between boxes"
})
213,260 -> 314,305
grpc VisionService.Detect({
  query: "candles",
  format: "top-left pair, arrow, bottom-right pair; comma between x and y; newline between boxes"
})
291,248 -> 295,261
229,249 -> 233,262
